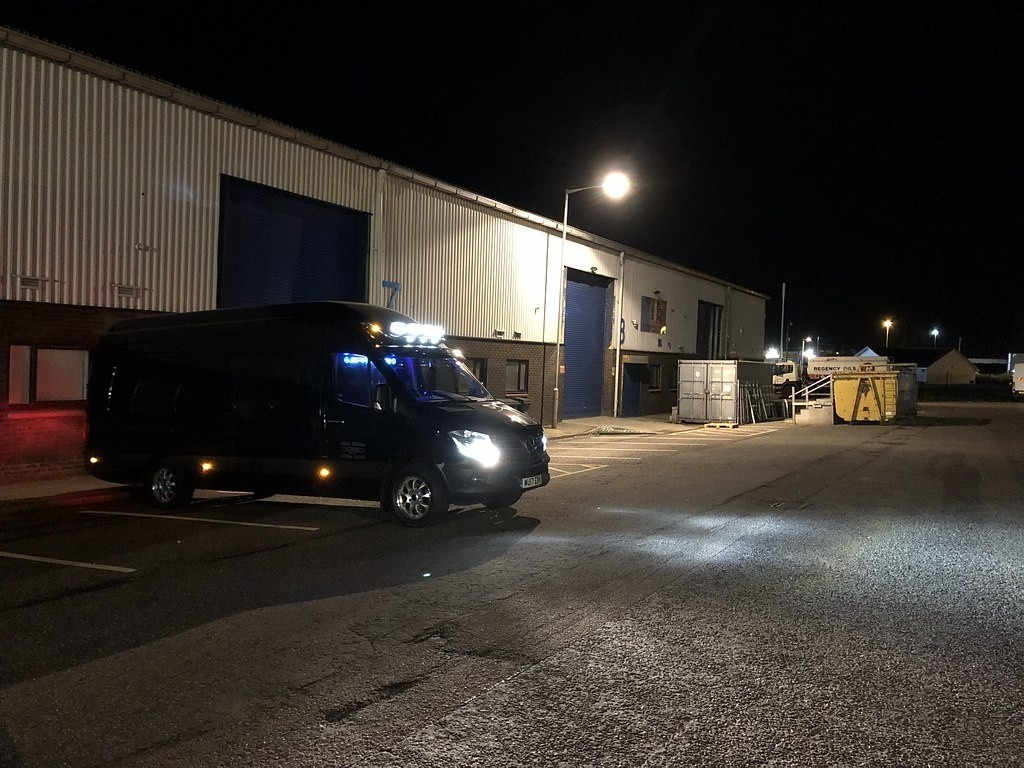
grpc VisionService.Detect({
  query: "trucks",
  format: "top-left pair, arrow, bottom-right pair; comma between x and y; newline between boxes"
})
772,356 -> 891,398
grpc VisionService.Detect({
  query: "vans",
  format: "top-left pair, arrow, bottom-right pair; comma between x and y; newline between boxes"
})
81,299 -> 551,529
1009,363 -> 1024,402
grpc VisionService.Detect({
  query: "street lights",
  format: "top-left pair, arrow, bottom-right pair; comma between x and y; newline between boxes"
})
932,329 -> 939,348
884,318 -> 892,349
551,170 -> 632,430
801,337 -> 810,371
